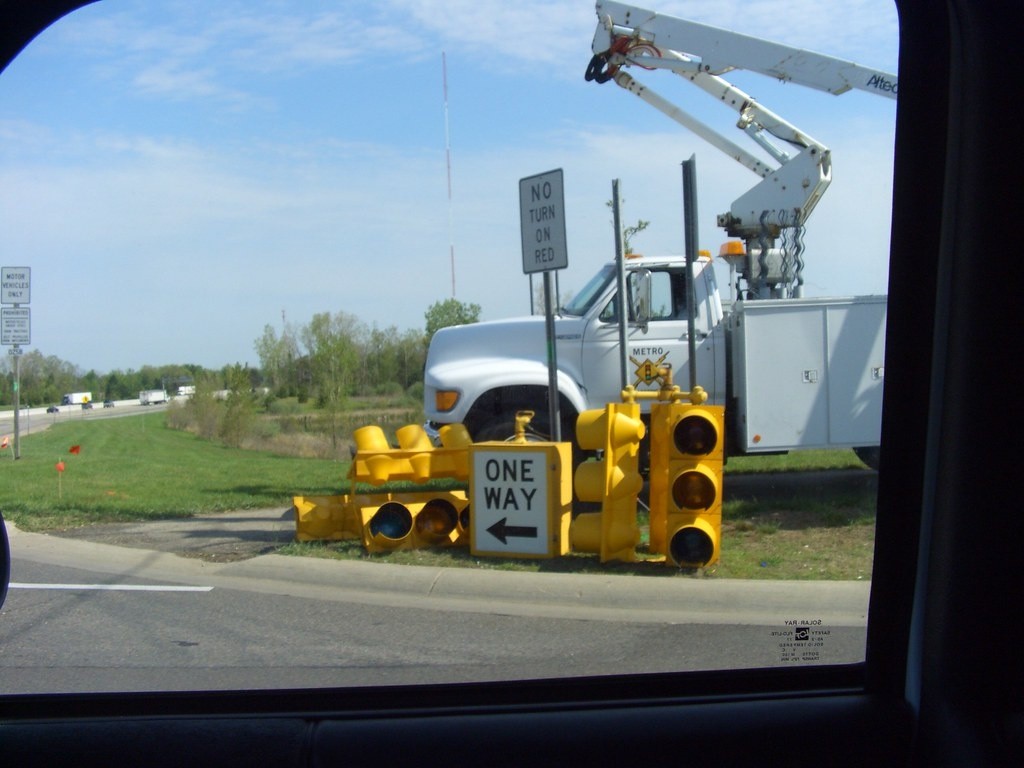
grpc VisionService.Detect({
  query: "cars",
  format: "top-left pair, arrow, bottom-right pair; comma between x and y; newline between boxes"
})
46,406 -> 59,414
82,403 -> 93,410
102,398 -> 114,409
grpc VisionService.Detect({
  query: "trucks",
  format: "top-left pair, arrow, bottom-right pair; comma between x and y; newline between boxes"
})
62,391 -> 93,406
177,385 -> 197,397
139,390 -> 170,406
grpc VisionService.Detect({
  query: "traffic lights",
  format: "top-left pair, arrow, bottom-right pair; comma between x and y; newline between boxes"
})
568,403 -> 645,565
649,403 -> 722,575
359,490 -> 474,556
345,426 -> 477,487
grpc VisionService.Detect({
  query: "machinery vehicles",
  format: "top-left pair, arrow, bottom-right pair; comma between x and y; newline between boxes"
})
422,2 -> 884,478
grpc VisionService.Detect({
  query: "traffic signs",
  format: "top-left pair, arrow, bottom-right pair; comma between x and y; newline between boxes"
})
474,450 -> 549,554
